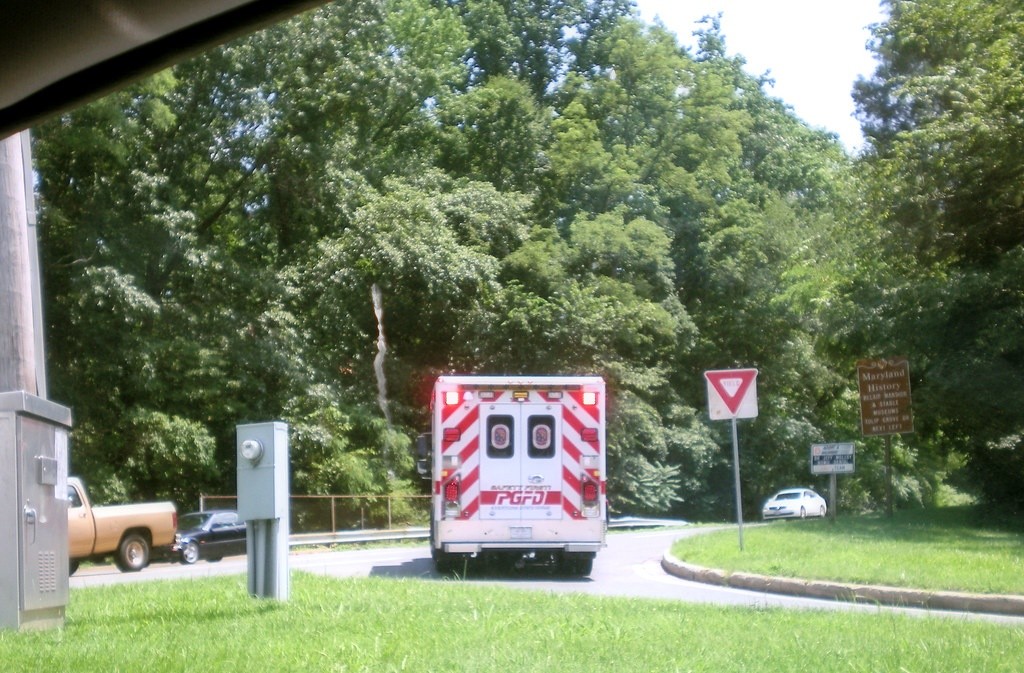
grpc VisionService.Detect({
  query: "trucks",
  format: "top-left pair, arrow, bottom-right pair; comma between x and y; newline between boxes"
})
418,372 -> 612,577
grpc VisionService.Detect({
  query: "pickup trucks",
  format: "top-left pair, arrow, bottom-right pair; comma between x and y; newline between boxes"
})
65,476 -> 176,578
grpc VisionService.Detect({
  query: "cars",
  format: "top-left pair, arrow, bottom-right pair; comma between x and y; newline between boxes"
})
762,488 -> 828,520
172,511 -> 246,566
